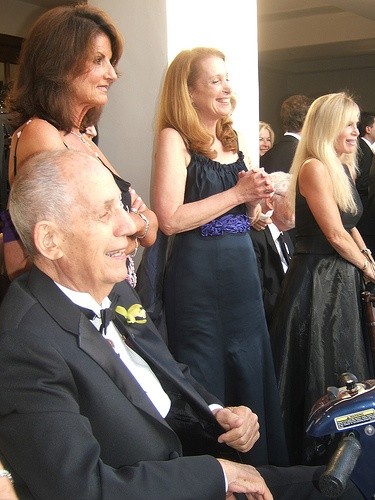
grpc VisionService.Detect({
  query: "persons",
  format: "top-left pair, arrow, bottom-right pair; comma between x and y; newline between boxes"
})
0,150 -> 375,499
2,7 -> 159,285
151,49 -> 287,465
247,92 -> 374,465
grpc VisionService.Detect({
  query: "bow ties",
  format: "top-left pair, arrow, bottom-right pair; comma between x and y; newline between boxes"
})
75,294 -> 120,335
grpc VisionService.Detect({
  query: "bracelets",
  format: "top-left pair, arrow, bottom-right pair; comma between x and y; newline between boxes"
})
362,257 -> 367,271
244,207 -> 259,218
360,249 -> 372,256
126,237 -> 138,257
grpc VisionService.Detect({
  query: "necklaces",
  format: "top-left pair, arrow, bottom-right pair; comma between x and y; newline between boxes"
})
67,128 -> 99,160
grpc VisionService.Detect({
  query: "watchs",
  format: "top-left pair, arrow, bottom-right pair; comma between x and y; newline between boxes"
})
134,213 -> 149,238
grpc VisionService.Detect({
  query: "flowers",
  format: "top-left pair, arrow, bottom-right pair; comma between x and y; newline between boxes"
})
114,304 -> 148,325
